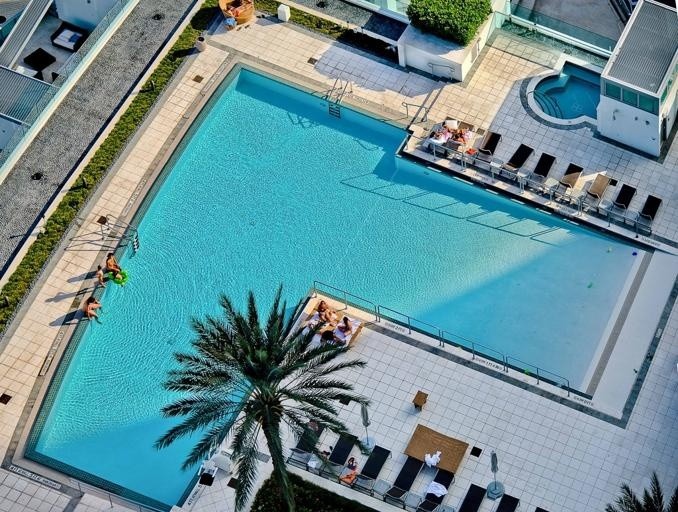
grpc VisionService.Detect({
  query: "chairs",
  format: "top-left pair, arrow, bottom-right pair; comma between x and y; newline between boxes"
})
213,450 -> 246,474
423,115 -> 662,235
198,461 -> 217,479
286,420 -> 551,512
218,0 -> 255,23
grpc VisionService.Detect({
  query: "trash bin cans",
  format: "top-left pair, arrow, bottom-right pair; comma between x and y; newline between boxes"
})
196,37 -> 206,51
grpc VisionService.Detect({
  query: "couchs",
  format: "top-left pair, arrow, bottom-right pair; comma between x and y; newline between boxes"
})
49,21 -> 88,53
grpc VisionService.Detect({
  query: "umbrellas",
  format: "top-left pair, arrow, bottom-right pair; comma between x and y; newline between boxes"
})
488,449 -> 500,490
360,401 -> 371,446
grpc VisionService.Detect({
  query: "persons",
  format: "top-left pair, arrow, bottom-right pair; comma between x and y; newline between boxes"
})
106,253 -> 121,272
96,264 -> 107,288
317,299 -> 334,322
111,271 -> 125,288
337,316 -> 352,337
453,127 -> 466,144
81,296 -> 104,325
225,3 -> 253,18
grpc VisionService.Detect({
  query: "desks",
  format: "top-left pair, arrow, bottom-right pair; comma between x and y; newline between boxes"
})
24,48 -> 57,72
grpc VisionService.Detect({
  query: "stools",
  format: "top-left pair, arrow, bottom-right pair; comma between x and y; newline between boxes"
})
221,20 -> 235,30
